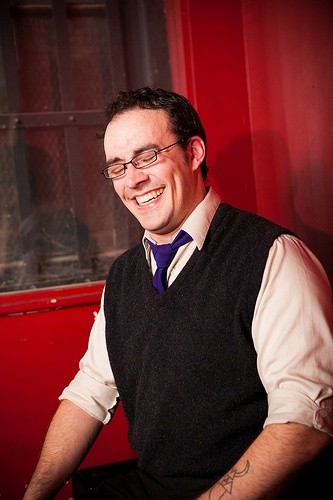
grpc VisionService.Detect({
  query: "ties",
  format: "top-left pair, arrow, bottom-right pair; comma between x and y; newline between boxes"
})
146,230 -> 193,291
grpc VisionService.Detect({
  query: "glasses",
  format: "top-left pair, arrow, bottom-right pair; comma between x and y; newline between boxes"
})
100,140 -> 183,179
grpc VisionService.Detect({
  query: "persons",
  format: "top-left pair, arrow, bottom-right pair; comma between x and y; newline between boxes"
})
20,88 -> 333,500
